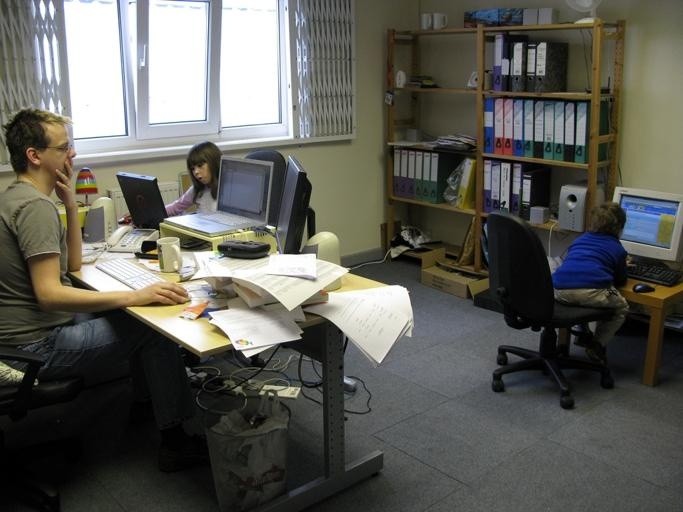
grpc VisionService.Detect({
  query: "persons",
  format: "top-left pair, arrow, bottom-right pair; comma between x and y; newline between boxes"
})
123,142 -> 224,224
0,108 -> 208,473
551,201 -> 630,365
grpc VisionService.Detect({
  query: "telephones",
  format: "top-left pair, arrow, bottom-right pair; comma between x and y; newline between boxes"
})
107,226 -> 159,253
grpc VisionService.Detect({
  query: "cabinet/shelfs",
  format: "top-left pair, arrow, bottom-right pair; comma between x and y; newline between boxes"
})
381,19 -> 625,286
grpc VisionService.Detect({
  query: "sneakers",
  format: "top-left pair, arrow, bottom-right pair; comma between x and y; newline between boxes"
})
575,331 -> 592,347
157,435 -> 209,471
585,347 -> 608,368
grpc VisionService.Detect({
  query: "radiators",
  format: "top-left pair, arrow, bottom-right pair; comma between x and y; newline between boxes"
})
108,180 -> 180,224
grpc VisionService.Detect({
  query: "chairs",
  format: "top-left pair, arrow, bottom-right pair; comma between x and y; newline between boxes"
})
0,346 -> 85,512
483,209 -> 620,411
242,146 -> 285,225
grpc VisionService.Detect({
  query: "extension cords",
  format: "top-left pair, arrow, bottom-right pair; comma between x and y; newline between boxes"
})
190,371 -> 243,396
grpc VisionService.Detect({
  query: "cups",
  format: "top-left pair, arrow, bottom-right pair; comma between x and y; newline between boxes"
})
432,13 -> 448,30
156,235 -> 180,273
418,14 -> 432,30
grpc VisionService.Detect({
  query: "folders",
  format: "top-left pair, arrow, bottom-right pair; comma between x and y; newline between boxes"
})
393,149 -> 464,204
483,97 -> 608,163
493,34 -> 568,92
484,160 -> 551,220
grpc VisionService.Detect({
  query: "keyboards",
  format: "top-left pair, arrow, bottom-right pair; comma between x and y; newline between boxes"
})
95,257 -> 169,291
626,264 -> 683,287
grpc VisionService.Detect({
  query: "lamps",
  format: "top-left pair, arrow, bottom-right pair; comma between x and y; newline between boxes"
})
75,165 -> 98,210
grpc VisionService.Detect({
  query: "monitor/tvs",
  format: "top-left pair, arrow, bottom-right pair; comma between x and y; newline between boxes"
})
612,187 -> 683,264
274,155 -> 317,256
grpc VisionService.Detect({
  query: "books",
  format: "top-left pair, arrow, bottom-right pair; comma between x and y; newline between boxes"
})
228,275 -> 343,311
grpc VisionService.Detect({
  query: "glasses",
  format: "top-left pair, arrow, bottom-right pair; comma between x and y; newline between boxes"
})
23,144 -> 73,154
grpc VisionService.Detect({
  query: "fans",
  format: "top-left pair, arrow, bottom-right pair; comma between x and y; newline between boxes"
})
561,0 -> 605,25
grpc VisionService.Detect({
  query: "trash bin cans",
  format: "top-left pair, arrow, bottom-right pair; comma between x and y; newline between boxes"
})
202,396 -> 291,512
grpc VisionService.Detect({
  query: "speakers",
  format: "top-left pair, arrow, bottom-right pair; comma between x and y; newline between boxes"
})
84,196 -> 117,243
558,180 -> 607,232
301,231 -> 341,292
530,207 -> 549,224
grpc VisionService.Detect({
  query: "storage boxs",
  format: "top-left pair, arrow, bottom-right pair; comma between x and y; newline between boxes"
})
419,245 -> 489,299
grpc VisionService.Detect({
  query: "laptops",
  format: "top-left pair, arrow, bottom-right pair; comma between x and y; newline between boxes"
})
117,172 -> 168,231
163,156 -> 274,237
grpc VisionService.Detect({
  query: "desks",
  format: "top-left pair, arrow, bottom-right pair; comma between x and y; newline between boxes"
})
68,228 -> 408,511
619,275 -> 683,389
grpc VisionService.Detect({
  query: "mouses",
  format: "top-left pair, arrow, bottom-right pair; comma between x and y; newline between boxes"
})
633,284 -> 655,293
118,216 -> 133,225
